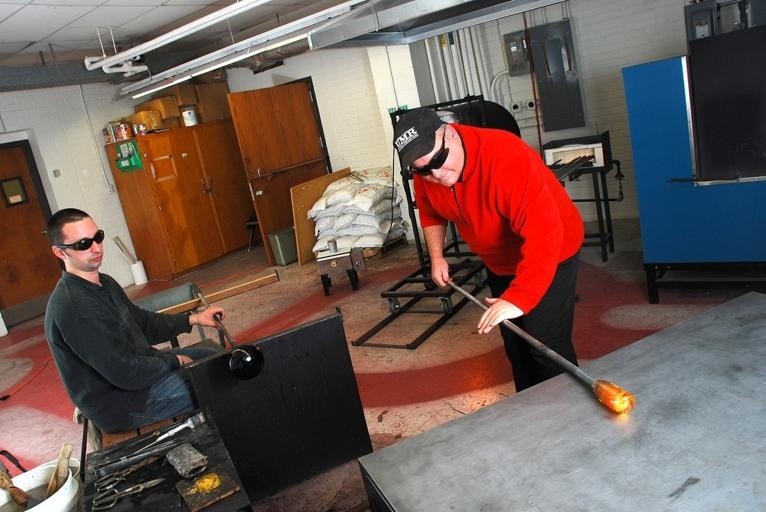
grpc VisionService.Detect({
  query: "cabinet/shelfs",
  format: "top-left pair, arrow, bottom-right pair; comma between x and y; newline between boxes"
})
100,116 -> 264,285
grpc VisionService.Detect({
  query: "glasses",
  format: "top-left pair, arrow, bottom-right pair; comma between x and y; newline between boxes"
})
50,230 -> 105,252
408,126 -> 450,178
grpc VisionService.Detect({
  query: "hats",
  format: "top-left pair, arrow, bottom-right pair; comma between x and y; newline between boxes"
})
393,108 -> 444,171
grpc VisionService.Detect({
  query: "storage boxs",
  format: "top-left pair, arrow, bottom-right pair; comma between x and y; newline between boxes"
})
194,72 -> 234,122
118,111 -> 164,132
159,81 -> 198,105
132,94 -> 182,118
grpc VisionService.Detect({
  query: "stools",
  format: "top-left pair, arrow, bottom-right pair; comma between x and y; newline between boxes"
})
245,218 -> 262,254
99,414 -> 195,452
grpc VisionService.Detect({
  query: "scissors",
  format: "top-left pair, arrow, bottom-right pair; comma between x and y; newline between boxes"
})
91,477 -> 166,511
94,457 -> 158,492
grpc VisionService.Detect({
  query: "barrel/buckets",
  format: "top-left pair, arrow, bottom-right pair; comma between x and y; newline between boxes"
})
0,457 -> 80,512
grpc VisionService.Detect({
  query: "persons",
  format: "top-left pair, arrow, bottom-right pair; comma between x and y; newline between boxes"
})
44,208 -> 224,434
394,109 -> 584,394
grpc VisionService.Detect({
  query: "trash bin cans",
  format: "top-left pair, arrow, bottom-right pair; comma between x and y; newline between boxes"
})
267,228 -> 298,266
